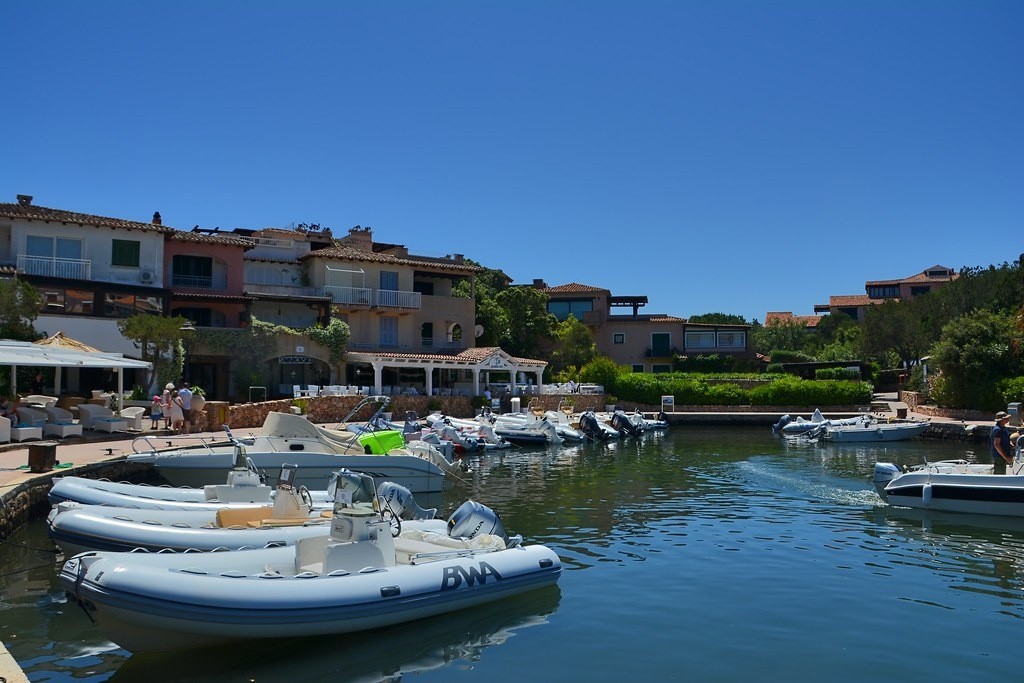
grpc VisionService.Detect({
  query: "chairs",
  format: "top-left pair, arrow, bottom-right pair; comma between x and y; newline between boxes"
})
293,383 -> 605,398
0,395 -> 146,443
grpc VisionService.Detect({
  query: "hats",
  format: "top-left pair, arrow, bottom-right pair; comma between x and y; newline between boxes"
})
995,411 -> 1011,422
153,395 -> 161,400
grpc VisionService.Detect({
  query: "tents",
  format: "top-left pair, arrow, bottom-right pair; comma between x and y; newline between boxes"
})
0,339 -> 152,419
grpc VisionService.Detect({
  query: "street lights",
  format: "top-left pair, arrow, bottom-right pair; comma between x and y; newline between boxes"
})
356,368 -> 361,395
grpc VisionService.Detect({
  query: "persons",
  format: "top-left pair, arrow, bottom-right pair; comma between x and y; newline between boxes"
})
991,411 -> 1015,475
179,383 -> 193,434
170,390 -> 185,434
162,383 -> 175,428
150,396 -> 165,429
483,387 -> 491,403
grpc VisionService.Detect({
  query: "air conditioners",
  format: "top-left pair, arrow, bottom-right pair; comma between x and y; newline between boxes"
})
140,271 -> 155,281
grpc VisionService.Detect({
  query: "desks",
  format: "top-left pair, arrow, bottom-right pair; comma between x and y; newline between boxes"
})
295,390 -> 317,397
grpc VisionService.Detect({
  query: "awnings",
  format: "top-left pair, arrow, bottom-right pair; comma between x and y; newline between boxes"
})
243,291 -> 332,309
172,291 -> 257,304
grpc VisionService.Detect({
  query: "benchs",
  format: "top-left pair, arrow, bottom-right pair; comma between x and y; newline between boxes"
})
393,538 -> 457,564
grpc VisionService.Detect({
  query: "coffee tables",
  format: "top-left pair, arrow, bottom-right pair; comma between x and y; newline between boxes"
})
11,426 -> 43,442
44,423 -> 82,438
95,418 -> 129,434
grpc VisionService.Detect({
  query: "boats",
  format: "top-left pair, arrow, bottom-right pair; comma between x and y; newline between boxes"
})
808,418 -> 930,443
47,500 -> 563,655
124,395 -> 469,517
771,408 -> 864,432
47,443 -> 437,564
299,493 -> 300,517
875,435 -> 1024,517
345,406 -> 670,464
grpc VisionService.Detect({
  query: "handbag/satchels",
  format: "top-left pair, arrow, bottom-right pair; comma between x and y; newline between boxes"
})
182,408 -> 186,416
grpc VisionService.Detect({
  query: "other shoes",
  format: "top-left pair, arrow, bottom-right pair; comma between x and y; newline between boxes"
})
168,422 -> 172,427
164,427 -> 168,429
150,427 -> 158,430
